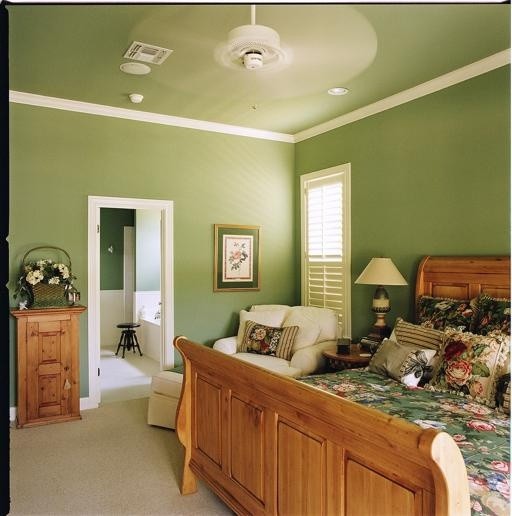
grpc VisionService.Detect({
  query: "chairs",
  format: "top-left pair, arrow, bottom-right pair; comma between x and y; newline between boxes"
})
212,304 -> 338,379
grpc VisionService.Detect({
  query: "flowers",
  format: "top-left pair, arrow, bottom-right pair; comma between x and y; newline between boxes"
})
15,257 -> 78,310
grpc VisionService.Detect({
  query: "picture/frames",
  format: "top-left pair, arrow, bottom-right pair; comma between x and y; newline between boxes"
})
213,224 -> 263,292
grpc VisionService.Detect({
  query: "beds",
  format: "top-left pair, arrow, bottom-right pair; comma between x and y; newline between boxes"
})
172,255 -> 510,516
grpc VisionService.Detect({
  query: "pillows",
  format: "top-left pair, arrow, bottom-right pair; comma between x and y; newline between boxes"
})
366,292 -> 510,416
281,310 -> 325,349
239,321 -> 299,363
236,308 -> 287,347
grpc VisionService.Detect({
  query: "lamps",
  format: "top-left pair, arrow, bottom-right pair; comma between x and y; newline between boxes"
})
353,255 -> 408,336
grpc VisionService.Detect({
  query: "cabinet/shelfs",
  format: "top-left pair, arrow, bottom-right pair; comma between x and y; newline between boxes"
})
10,304 -> 83,429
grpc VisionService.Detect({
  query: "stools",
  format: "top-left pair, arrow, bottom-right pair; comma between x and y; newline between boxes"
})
147,364 -> 185,431
115,323 -> 144,359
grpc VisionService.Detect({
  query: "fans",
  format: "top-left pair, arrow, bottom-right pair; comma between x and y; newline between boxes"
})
128,4 -> 377,99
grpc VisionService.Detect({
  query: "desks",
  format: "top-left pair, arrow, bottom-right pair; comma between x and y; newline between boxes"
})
321,343 -> 371,368
